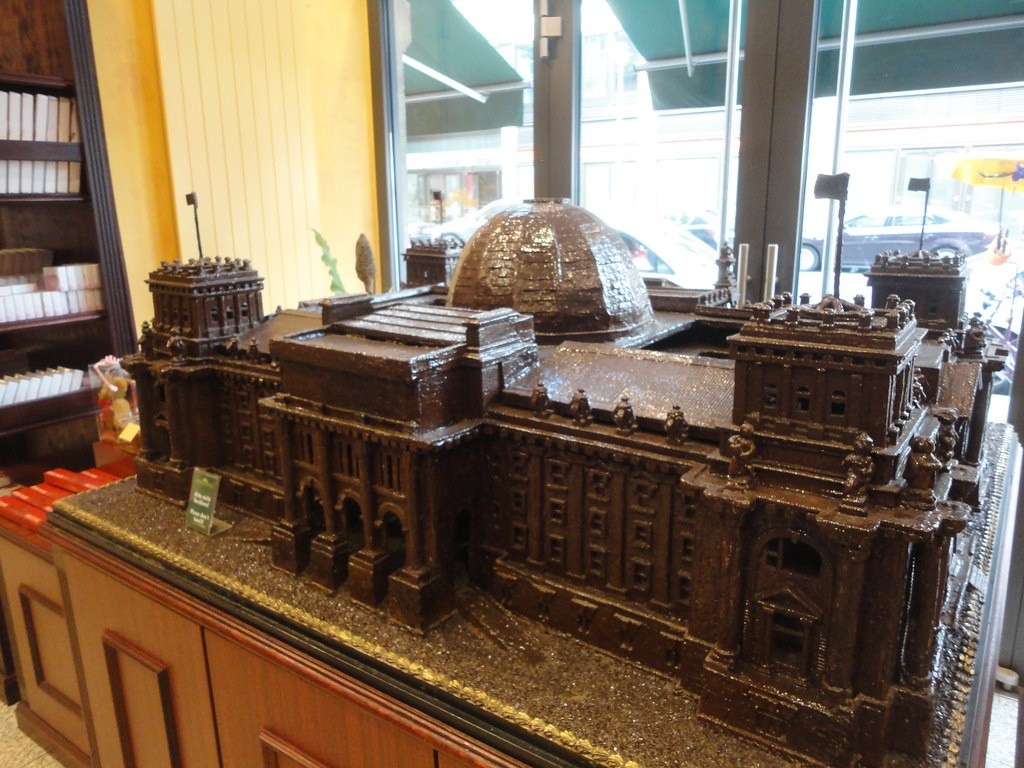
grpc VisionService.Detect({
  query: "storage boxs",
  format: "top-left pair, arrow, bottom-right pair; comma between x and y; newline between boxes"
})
0,453 -> 135,530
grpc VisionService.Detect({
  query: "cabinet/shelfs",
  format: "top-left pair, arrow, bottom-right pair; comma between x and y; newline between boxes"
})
1,0 -> 140,438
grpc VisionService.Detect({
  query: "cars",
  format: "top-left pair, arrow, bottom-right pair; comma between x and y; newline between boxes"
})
799,205 -> 999,271
606,222 -> 719,288
429,199 -> 520,261
